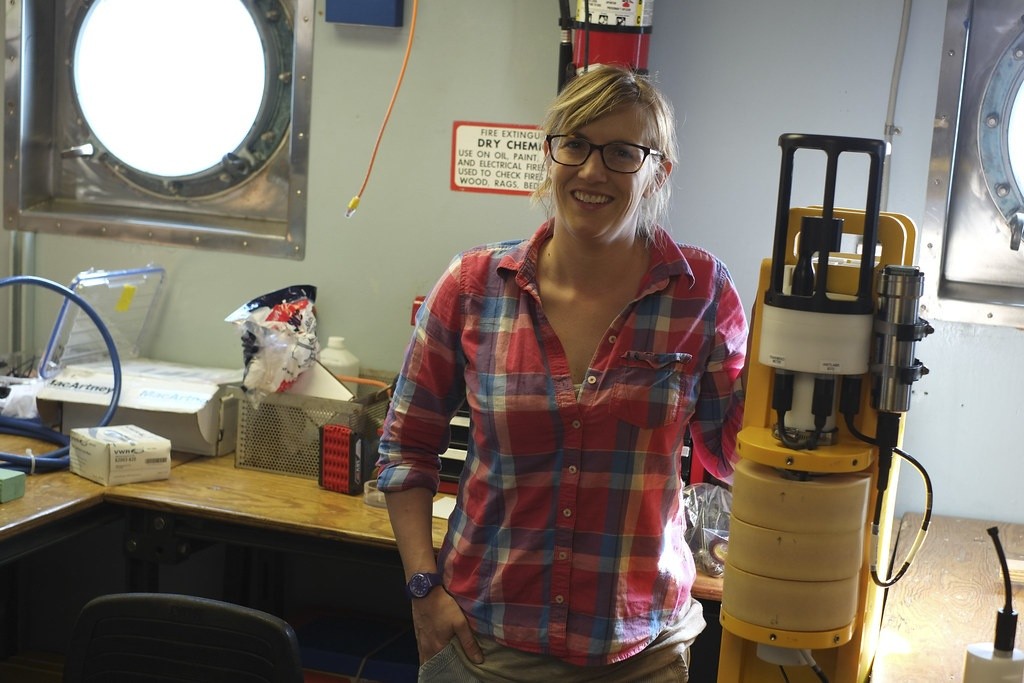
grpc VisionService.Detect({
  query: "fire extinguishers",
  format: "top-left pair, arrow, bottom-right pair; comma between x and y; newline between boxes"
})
556,0 -> 654,95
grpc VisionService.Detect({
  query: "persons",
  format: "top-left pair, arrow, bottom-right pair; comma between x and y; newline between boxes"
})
375,65 -> 757,683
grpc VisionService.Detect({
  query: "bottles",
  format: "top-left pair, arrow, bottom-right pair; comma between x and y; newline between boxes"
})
316,337 -> 358,399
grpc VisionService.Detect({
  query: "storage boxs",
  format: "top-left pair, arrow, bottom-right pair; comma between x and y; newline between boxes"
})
0,468 -> 26,503
224,368 -> 400,482
69,424 -> 172,485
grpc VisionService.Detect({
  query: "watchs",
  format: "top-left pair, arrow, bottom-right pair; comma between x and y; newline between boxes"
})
407,572 -> 443,599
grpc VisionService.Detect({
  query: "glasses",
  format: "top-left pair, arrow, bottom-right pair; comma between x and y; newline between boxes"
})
546,135 -> 665,174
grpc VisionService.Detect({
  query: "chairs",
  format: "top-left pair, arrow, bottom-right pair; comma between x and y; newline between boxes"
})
63,592 -> 304,683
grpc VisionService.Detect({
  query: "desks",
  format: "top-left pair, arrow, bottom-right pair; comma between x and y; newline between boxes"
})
0,430 -> 723,683
